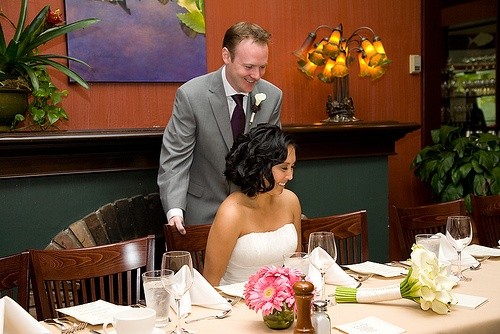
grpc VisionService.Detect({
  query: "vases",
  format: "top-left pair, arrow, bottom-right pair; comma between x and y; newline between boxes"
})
0,87 -> 33,132
263,302 -> 294,330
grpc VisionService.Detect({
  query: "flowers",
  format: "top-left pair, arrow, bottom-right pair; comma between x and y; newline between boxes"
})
334,243 -> 460,314
243,265 -> 318,320
0,0 -> 102,134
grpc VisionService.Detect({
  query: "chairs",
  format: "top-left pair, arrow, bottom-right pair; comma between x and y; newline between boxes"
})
163,224 -> 212,276
0,251 -> 31,312
470,193 -> 500,248
301,209 -> 369,270
27,235 -> 156,321
390,199 -> 467,259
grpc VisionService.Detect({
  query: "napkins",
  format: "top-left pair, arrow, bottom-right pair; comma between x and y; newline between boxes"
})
171,265 -> 232,315
307,246 -> 356,286
430,233 -> 478,267
0,296 -> 52,334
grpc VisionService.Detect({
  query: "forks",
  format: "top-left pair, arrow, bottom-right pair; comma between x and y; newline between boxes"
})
58,317 -> 87,331
44,319 -> 76,334
185,309 -> 231,324
470,263 -> 483,270
231,296 -> 242,306
475,255 -> 490,262
349,273 -> 374,282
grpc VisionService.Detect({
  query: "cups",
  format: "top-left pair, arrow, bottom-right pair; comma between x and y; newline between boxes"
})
103,308 -> 156,334
142,270 -> 174,328
416,234 -> 441,263
283,252 -> 311,282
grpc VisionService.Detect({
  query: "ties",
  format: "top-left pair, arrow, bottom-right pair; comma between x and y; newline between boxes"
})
230,94 -> 246,141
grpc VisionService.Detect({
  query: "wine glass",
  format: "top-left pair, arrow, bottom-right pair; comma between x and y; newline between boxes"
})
446,216 -> 473,281
307,232 -> 338,305
160,251 -> 194,334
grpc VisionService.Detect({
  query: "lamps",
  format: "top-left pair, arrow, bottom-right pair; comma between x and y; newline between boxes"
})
291,23 -> 393,122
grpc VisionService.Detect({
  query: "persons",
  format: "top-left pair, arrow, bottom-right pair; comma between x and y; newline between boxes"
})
157,20 -> 283,282
195,122 -> 303,296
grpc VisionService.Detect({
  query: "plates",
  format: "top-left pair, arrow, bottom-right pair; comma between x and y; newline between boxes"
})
215,281 -> 247,300
341,261 -> 405,277
468,244 -> 500,258
55,299 -> 123,326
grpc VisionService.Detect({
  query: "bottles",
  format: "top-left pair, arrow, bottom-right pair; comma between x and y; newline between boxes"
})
292,274 -> 315,334
472,78 -> 496,96
462,53 -> 496,71
312,300 -> 331,334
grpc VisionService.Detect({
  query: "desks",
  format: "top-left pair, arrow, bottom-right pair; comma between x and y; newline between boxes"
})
40,257 -> 500,334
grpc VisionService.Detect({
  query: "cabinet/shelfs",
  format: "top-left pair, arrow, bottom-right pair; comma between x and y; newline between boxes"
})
434,62 -> 497,99
